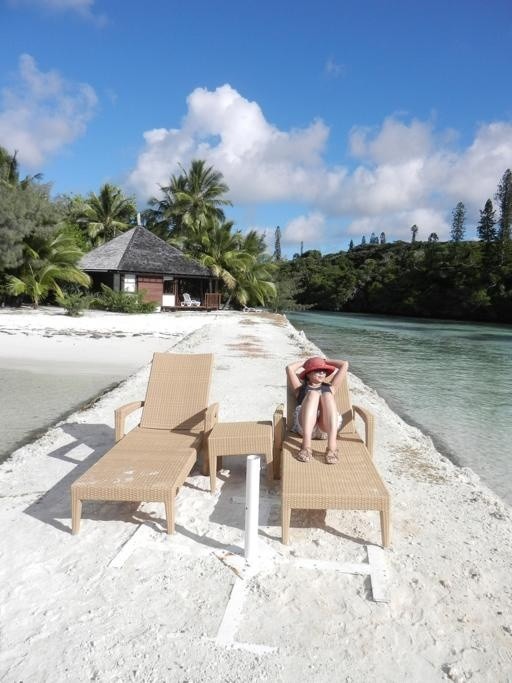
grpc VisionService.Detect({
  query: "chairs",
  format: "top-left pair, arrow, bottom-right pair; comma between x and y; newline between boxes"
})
70,351 -> 219,534
272,373 -> 391,548
182,292 -> 201,306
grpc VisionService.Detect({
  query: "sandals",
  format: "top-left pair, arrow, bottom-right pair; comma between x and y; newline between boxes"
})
324,447 -> 340,463
297,445 -> 313,462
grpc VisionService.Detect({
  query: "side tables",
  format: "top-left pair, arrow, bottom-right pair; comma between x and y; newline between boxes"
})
207,420 -> 273,493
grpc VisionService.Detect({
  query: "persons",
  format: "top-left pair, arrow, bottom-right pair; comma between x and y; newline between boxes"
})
285,354 -> 350,465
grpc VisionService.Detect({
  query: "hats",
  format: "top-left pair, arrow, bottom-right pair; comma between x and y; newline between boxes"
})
298,357 -> 336,381
